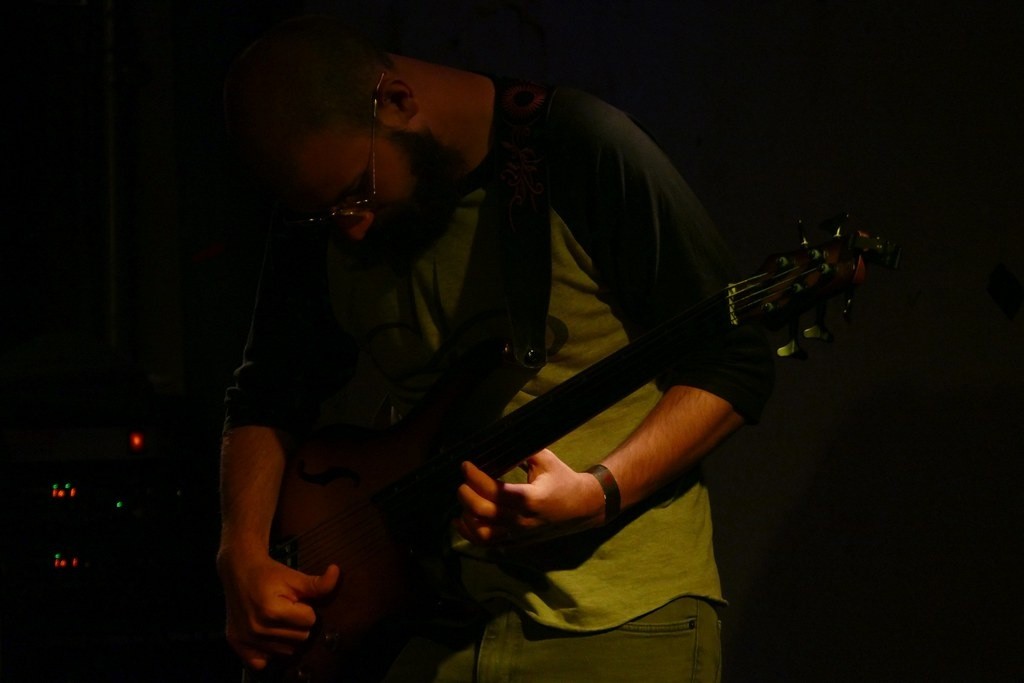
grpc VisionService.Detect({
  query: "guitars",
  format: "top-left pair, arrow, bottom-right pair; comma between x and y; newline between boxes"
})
243,209 -> 900,683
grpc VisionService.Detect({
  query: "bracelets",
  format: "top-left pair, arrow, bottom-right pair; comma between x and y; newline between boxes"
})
582,465 -> 621,525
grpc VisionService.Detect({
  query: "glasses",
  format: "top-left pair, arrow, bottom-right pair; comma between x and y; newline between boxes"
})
282,71 -> 386,229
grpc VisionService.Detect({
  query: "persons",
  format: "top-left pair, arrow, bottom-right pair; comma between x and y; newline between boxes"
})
217,1 -> 777,682
